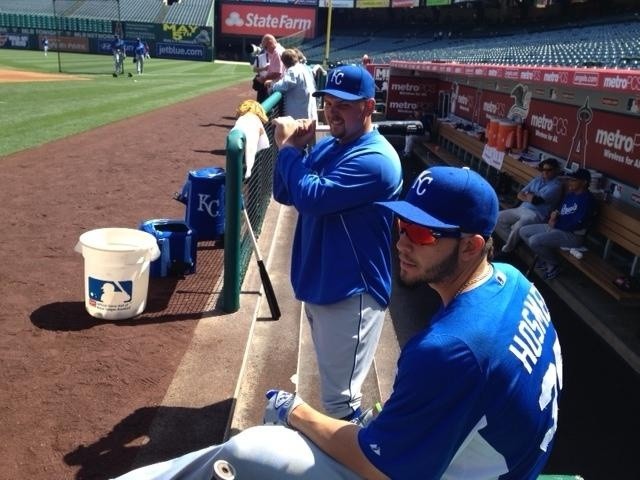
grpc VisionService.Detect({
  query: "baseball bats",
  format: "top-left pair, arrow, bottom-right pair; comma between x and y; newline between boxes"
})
315,121 -> 423,135
239,194 -> 281,320
525,210 -> 559,278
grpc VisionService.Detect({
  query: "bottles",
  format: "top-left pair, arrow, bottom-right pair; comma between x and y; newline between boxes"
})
487,119 -> 517,152
602,188 -> 612,202
516,124 -> 529,149
359,402 -> 382,428
459,120 -> 464,128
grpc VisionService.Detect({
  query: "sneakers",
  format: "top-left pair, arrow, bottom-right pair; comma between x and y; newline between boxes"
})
502,244 -> 514,253
535,259 -> 562,280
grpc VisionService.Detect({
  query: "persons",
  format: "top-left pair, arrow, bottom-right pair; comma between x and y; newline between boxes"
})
270,62 -> 407,423
517,168 -> 593,282
493,157 -> 564,253
168,164 -> 569,480
111,34 -> 126,74
42,36 -> 48,56
247,33 -> 320,155
133,37 -> 147,74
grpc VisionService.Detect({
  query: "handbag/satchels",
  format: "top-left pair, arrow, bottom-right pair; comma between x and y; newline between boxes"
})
252,75 -> 263,90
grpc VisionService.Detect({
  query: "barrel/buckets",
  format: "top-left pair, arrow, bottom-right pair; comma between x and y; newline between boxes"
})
496,120 -> 517,151
73,227 -> 162,321
487,119 -> 507,147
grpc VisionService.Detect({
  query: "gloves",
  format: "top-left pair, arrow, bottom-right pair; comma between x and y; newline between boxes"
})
262,390 -> 305,425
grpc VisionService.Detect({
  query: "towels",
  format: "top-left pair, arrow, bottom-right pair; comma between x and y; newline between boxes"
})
482,144 -> 496,165
490,151 -> 504,170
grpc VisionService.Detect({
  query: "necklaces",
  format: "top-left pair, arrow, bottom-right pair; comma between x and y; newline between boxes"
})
453,264 -> 494,299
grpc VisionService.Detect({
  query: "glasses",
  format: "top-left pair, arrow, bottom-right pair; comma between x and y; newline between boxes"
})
543,167 -> 554,171
397,217 -> 461,246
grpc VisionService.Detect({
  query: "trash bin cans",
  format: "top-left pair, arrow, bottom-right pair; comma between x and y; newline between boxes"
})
182,168 -> 226,241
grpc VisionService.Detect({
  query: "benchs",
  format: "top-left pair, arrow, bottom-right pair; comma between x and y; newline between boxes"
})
419,119 -> 640,307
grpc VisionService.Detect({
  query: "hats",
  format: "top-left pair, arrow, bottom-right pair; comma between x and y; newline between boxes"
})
311,64 -> 376,102
566,168 -> 591,182
372,164 -> 501,240
